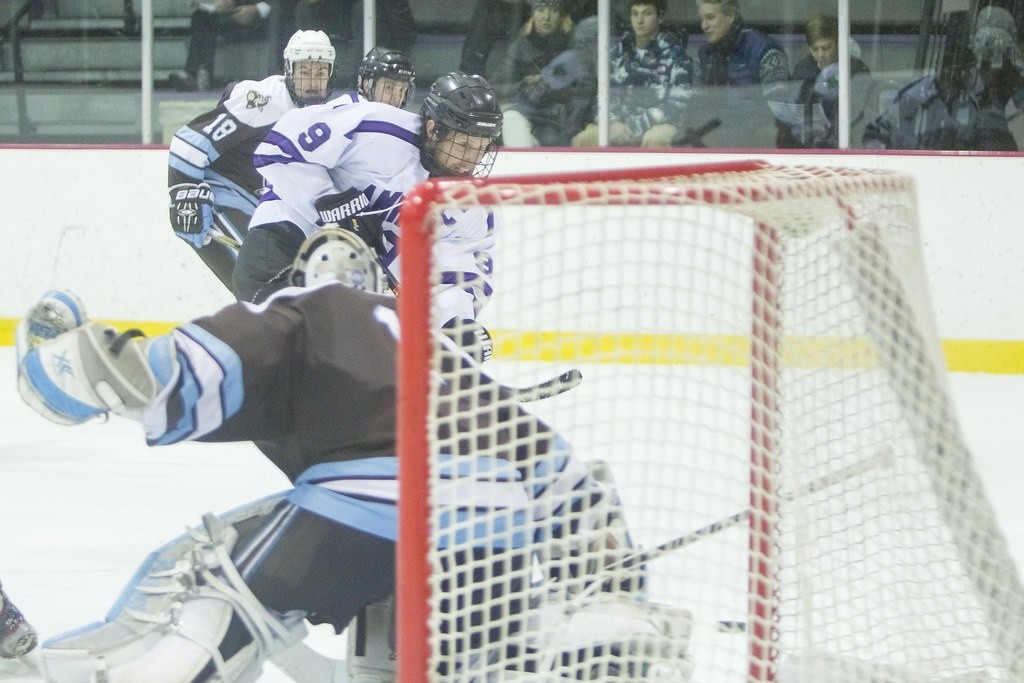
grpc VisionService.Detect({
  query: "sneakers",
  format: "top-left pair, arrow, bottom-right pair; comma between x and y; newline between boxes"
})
1,585 -> 37,669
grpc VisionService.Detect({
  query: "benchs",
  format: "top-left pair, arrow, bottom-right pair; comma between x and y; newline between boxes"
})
0,0 -> 949,151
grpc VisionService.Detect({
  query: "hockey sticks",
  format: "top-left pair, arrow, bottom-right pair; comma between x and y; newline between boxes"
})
526,445 -> 909,610
345,204 -> 586,407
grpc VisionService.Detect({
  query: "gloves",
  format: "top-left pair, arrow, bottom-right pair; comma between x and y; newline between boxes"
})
608,122 -> 634,146
639,123 -> 678,149
168,183 -> 214,249
314,186 -> 387,258
439,317 -> 493,375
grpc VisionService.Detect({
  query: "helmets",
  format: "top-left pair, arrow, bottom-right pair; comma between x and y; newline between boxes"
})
356,45 -> 416,111
289,223 -> 385,307
283,29 -> 336,107
415,71 -> 504,178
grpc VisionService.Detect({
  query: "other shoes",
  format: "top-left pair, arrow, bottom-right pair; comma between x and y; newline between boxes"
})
170,70 -> 196,91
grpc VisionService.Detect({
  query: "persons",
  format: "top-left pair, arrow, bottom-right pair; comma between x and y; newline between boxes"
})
13,279 -> 696,681
166,28 -> 336,302
166,0 -> 1024,156
322,45 -> 417,110
233,70 -> 506,360
0,586 -> 40,673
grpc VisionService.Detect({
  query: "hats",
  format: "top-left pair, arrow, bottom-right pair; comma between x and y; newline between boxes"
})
528,0 -> 565,11
